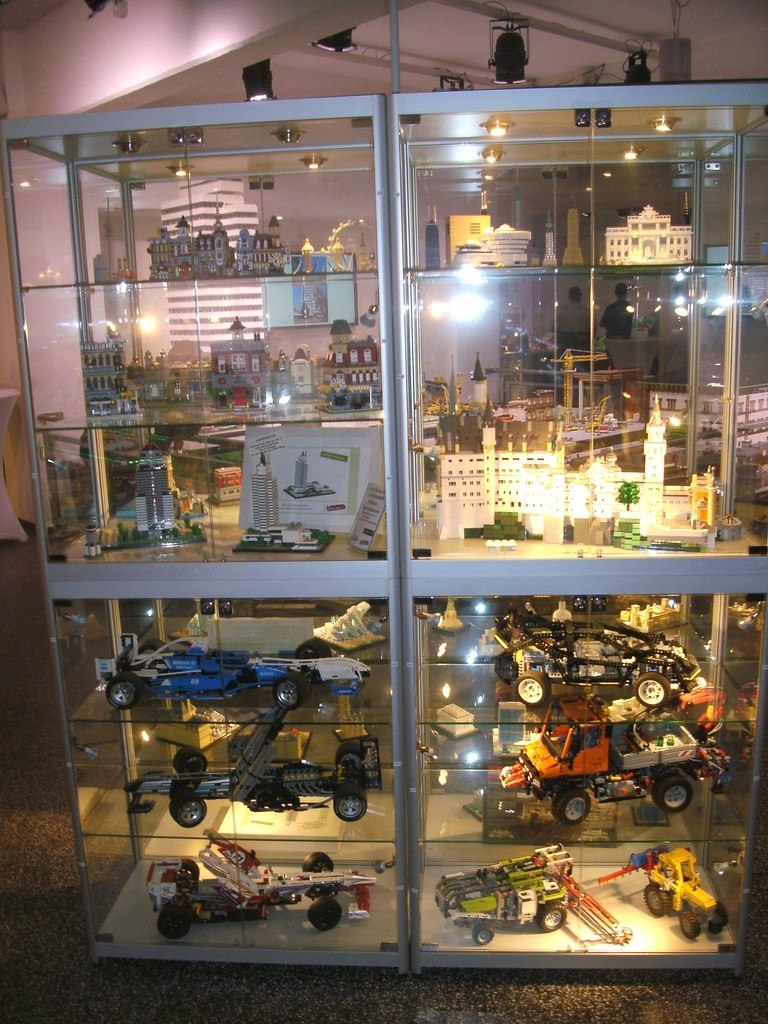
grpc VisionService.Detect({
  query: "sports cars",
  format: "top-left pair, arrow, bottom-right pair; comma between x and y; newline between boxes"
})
489,602 -> 703,710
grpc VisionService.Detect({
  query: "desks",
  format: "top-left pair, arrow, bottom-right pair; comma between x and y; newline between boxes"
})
605,336 -> 663,375
0,389 -> 28,542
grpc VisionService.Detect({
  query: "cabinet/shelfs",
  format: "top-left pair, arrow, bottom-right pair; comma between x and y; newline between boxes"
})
0,80 -> 409,976
385,82 -> 768,977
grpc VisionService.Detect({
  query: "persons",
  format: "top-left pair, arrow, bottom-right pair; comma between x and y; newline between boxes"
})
551,285 -> 593,367
80,340 -> 210,521
599,281 -> 633,369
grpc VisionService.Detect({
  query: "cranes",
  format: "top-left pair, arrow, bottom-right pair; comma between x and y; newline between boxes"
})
536,346 -> 611,432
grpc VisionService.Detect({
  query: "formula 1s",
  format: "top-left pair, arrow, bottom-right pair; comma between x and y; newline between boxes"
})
123,706 -> 382,827
105,633 -> 375,711
147,826 -> 378,939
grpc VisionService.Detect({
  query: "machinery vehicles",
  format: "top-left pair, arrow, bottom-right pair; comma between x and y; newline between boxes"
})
596,843 -> 726,938
584,394 -> 611,434
498,682 -> 727,826
435,842 -> 575,946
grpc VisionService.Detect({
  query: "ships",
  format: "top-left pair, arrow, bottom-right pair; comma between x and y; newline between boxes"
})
448,229 -> 532,269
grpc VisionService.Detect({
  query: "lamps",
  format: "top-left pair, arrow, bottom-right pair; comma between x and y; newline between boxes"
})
311,27 -> 357,53
488,17 -> 530,84
242,58 -> 279,102
623,52 -> 651,83
